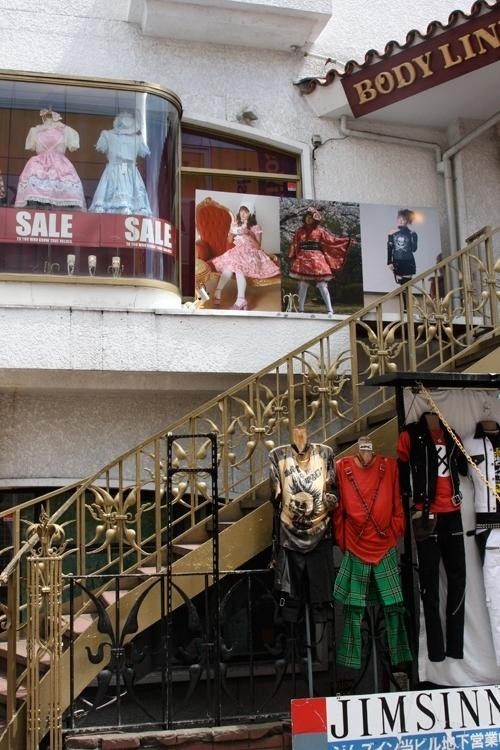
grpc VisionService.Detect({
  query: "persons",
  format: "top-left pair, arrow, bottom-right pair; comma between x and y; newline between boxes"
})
464,420 -> 499,670
396,413 -> 468,662
387,209 -> 418,313
88,111 -> 152,277
288,206 -> 351,313
330,435 -> 414,670
268,427 -> 335,619
212,201 -> 281,310
15,108 -> 88,273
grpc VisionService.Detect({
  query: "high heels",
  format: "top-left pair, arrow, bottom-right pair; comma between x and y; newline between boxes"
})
230,297 -> 248,310
214,288 -> 222,305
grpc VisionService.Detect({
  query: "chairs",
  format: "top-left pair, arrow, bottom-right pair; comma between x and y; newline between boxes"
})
195,196 -> 281,287
